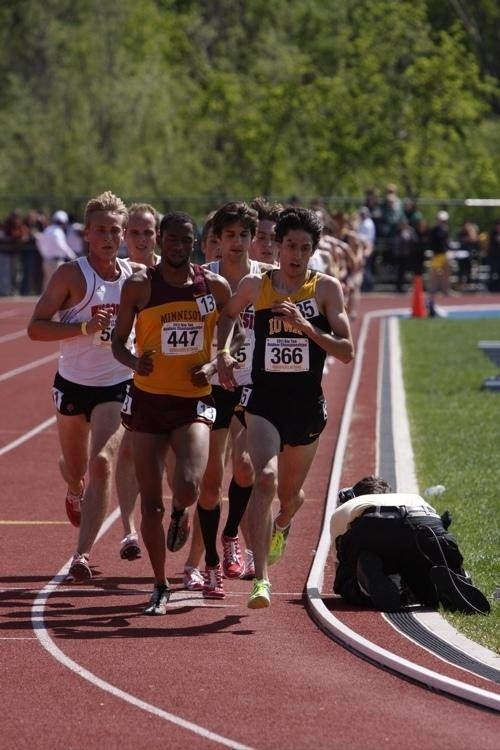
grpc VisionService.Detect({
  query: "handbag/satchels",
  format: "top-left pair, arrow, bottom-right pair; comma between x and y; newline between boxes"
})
216,348 -> 230,355
80,320 -> 88,336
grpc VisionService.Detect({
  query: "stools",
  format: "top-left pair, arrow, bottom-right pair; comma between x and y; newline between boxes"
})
360,505 -> 439,517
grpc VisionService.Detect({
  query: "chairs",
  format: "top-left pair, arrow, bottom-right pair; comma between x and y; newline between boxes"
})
143,579 -> 170,616
247,578 -> 272,609
240,548 -> 256,581
357,550 -> 401,612
65,477 -> 86,528
69,551 -> 92,583
268,515 -> 292,565
184,566 -> 206,591
221,528 -> 242,577
202,556 -> 225,598
120,537 -> 143,561
430,566 -> 491,618
167,496 -> 191,552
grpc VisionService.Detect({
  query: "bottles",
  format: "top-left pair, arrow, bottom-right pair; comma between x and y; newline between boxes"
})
52,210 -> 69,224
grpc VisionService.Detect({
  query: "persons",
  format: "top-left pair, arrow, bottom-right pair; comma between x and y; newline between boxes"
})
28,191 -> 152,581
354,181 -> 499,298
216,207 -> 355,609
111,211 -> 246,617
303,208 -> 354,289
0,208 -> 37,296
182,210 -> 224,594
193,200 -> 280,600
241,197 -> 284,581
114,204 -> 177,560
37,209 -> 78,292
